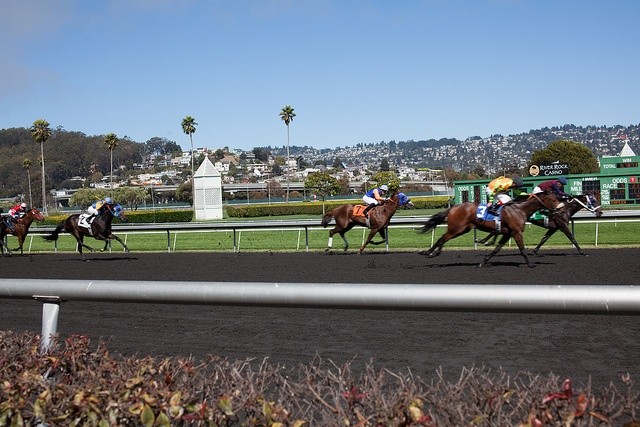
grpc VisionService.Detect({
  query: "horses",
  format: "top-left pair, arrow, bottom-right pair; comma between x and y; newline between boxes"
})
41,201 -> 130,260
320,188 -> 415,255
415,189 -> 566,268
476,191 -> 603,256
0,208 -> 45,256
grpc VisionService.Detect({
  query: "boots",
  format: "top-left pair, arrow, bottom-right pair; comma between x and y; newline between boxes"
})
487,203 -> 499,216
363,203 -> 375,219
86,214 -> 95,222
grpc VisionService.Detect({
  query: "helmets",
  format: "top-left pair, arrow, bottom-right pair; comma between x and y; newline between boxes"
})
559,178 -> 567,185
106,198 -> 113,204
513,178 -> 523,186
379,185 -> 388,191
21,203 -> 27,208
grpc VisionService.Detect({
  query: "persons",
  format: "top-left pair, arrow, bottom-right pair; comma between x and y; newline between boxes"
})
533,178 -> 571,209
486,176 -> 522,216
5,203 -> 27,227
362,185 -> 387,217
86,198 -> 112,222
